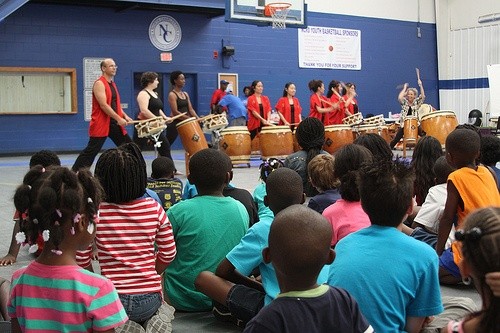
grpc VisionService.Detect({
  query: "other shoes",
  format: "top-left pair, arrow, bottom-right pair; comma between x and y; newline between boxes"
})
145,300 -> 175,333
114,320 -> 145,333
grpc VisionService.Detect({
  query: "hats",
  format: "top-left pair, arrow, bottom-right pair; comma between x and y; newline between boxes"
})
225,88 -> 234,94
220,79 -> 229,84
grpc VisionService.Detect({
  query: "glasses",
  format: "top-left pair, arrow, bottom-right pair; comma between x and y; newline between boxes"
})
103,65 -> 119,69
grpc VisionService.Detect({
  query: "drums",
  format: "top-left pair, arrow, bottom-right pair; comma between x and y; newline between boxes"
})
389,124 -> 403,146
421,110 -> 459,150
200,114 -> 229,133
257,126 -> 295,162
352,126 -> 389,145
175,117 -> 208,158
220,127 -> 252,164
342,112 -> 364,126
134,116 -> 167,139
404,116 -> 418,146
292,126 -> 303,151
418,104 -> 437,122
364,114 -> 385,125
324,125 -> 353,153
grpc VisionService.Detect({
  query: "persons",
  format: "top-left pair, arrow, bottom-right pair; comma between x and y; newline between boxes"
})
211,79 -> 358,141
0,118 -> 500,333
72,59 -> 134,174
165,71 -> 201,143
135,73 -> 184,175
391,79 -> 426,149
